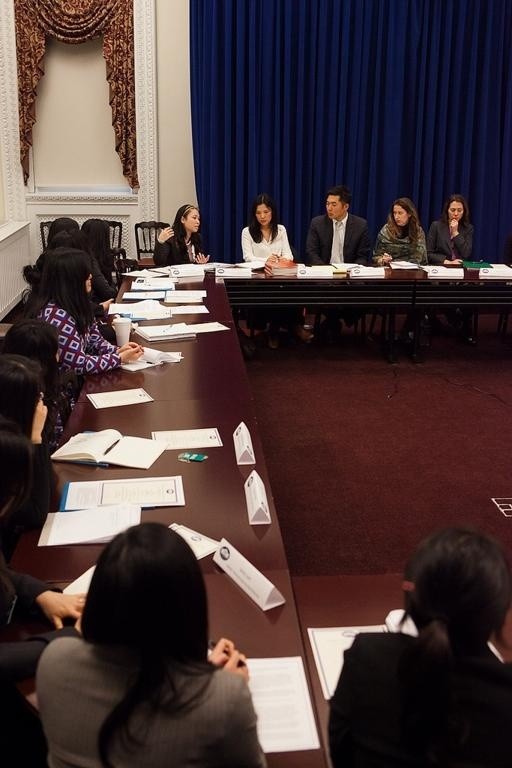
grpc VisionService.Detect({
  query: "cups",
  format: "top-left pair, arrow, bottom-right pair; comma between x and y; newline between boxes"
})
111,317 -> 132,346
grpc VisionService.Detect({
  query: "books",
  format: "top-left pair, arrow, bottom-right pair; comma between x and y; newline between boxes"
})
117,345 -> 184,371
463,260 -> 493,270
311,262 -> 365,274
384,608 -> 503,664
134,322 -> 197,342
389,259 -> 419,271
49,427 -> 170,470
107,260 -> 312,322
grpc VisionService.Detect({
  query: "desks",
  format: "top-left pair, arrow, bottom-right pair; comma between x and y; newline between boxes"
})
0,266 -> 326,767
292,574 -> 512,768
223,260 -> 512,363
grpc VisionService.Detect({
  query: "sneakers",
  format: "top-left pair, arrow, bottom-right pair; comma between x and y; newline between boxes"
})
234,317 -> 480,351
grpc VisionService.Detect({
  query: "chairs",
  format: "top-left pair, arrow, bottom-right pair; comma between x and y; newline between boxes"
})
107,221 -> 122,248
110,248 -> 126,261
248,309 -> 508,340
134,221 -> 170,269
40,221 -> 53,253
114,259 -> 139,289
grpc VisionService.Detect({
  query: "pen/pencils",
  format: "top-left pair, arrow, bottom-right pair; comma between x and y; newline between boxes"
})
208,639 -> 245,667
137,360 -> 155,364
103,439 -> 120,454
380,253 -> 393,260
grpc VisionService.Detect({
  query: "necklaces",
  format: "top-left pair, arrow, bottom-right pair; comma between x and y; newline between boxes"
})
186,240 -> 192,245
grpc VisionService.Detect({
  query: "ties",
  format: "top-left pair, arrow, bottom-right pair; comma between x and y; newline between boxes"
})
333,222 -> 343,265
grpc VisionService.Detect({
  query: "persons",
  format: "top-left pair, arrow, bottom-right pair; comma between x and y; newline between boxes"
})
426,192 -> 478,347
1,321 -> 87,677
240,192 -> 315,349
24,216 -> 145,439
326,520 -> 512,768
152,203 -> 239,326
370,197 -> 429,346
306,184 -> 373,345
33,519 -> 267,768
